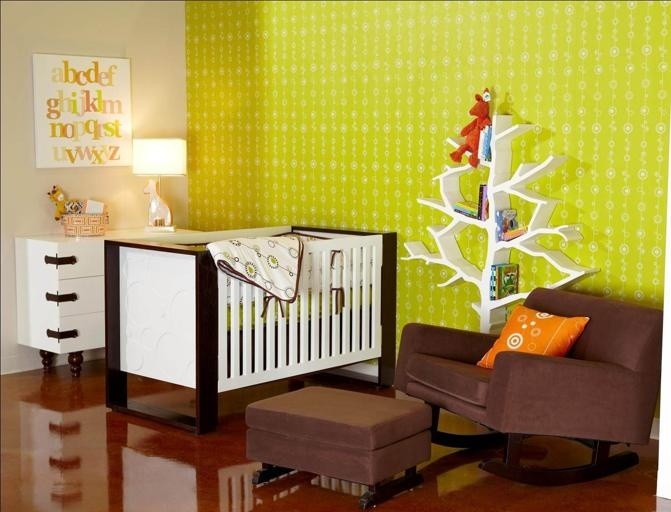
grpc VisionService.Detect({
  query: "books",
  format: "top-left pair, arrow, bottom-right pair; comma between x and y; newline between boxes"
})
494,208 -> 528,241
489,263 -> 518,301
476,125 -> 491,162
453,184 -> 489,222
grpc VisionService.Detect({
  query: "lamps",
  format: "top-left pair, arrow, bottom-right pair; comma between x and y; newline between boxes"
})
132,137 -> 187,229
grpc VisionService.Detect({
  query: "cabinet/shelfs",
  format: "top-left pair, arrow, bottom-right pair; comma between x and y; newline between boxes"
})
398,113 -> 599,334
15,228 -> 204,379
17,373 -> 192,512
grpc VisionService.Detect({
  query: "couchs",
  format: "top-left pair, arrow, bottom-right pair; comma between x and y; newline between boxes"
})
393,288 -> 661,483
244,386 -> 433,507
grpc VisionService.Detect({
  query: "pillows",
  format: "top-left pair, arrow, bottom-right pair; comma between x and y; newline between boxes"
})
478,305 -> 588,370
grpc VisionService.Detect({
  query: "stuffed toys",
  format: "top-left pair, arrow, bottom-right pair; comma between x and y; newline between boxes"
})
450,88 -> 491,167
46,185 -> 85,221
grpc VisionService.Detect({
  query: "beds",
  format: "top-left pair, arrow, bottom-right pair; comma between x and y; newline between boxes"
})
103,412 -> 421,512
102,225 -> 396,434
